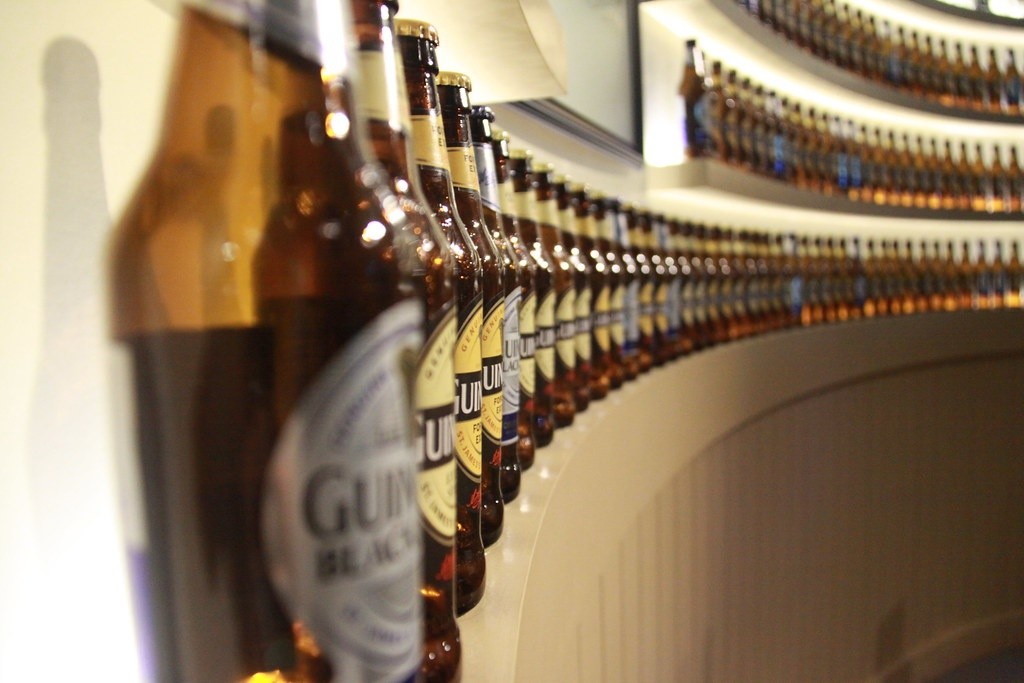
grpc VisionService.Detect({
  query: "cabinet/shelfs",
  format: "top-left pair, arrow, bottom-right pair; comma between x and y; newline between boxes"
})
388,0 -> 1024,227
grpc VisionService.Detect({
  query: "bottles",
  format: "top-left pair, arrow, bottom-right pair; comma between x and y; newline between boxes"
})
109,0 -> 1024,683
